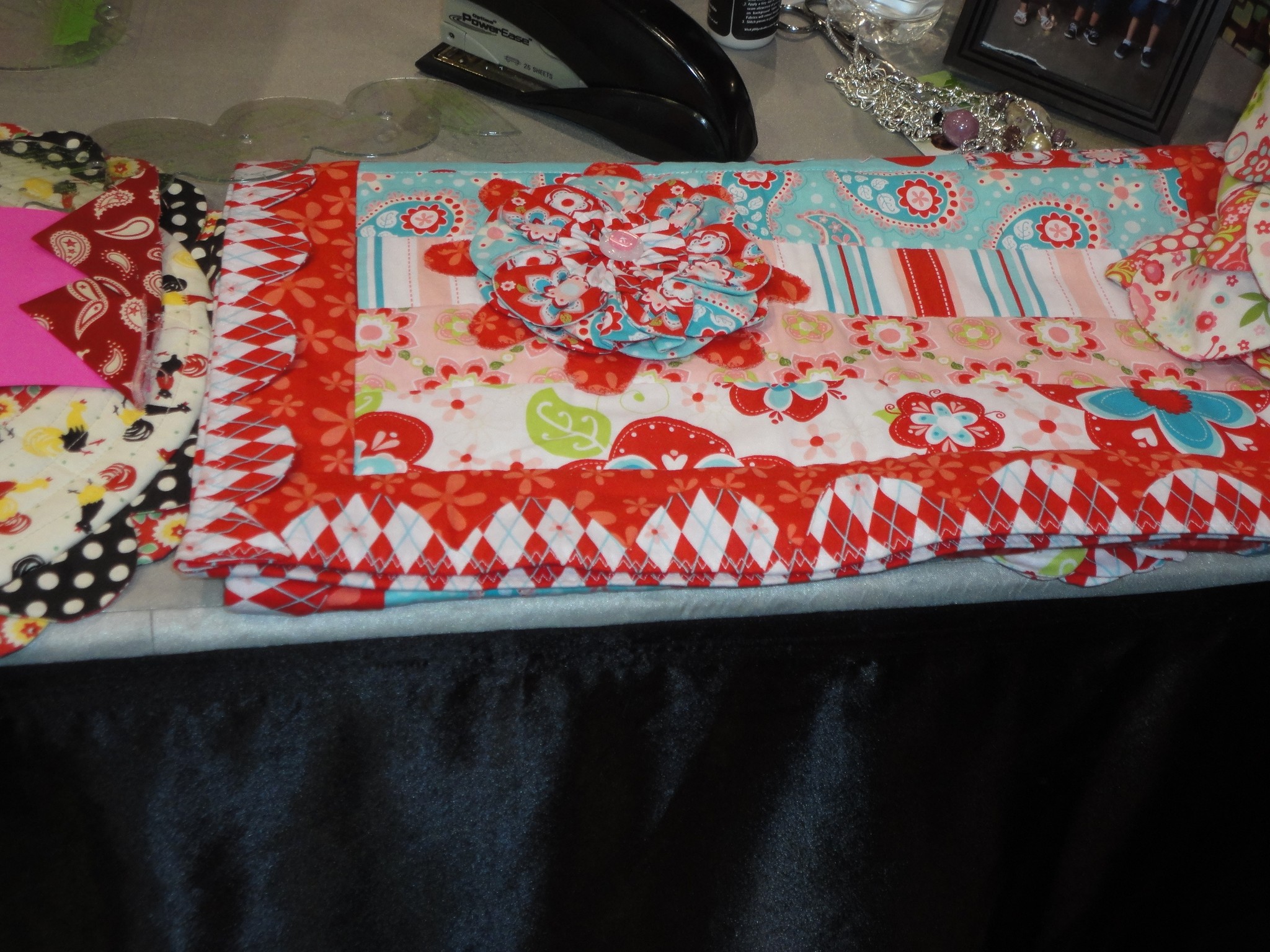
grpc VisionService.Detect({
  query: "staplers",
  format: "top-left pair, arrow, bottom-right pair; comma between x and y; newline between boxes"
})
414,0 -> 761,168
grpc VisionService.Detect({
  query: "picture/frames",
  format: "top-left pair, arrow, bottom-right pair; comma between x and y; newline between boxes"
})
935,2 -> 1240,155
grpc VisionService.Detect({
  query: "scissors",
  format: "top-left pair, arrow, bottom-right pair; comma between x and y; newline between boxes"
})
774,0 -> 891,80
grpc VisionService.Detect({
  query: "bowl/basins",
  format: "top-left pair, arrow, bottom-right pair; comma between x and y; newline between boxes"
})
827,0 -> 945,44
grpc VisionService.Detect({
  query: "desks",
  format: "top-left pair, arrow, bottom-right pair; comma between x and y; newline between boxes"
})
0,0 -> 1269,952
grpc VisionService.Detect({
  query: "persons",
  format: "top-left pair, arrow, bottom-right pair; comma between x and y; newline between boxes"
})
1014,0 -> 1055,31
1063,0 -> 1109,46
1113,0 -> 1170,70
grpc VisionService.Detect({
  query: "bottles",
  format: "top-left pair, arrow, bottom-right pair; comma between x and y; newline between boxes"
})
705,1 -> 781,51
